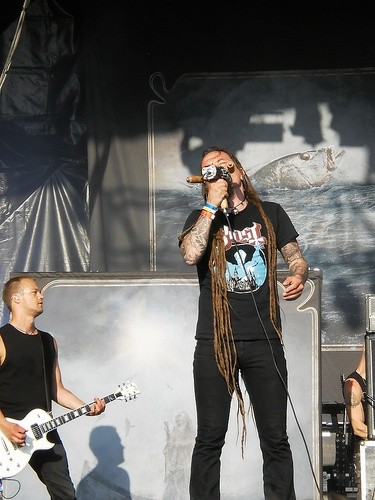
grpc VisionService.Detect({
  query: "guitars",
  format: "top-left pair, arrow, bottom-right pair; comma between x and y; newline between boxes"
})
0,380 -> 141,480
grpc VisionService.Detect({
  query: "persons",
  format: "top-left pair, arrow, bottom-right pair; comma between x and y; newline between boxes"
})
0,277 -> 106,500
342,337 -> 375,499
177,147 -> 310,500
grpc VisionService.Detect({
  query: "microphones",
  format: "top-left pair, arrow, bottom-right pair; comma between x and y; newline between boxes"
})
201,164 -> 232,216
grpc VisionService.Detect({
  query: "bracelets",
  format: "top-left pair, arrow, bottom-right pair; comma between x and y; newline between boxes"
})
201,202 -> 218,219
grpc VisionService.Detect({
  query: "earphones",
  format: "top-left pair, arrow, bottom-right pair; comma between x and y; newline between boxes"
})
16,297 -> 18,300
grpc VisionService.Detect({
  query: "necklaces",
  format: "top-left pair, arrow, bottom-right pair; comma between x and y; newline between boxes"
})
228,197 -> 246,215
12,324 -> 36,336
